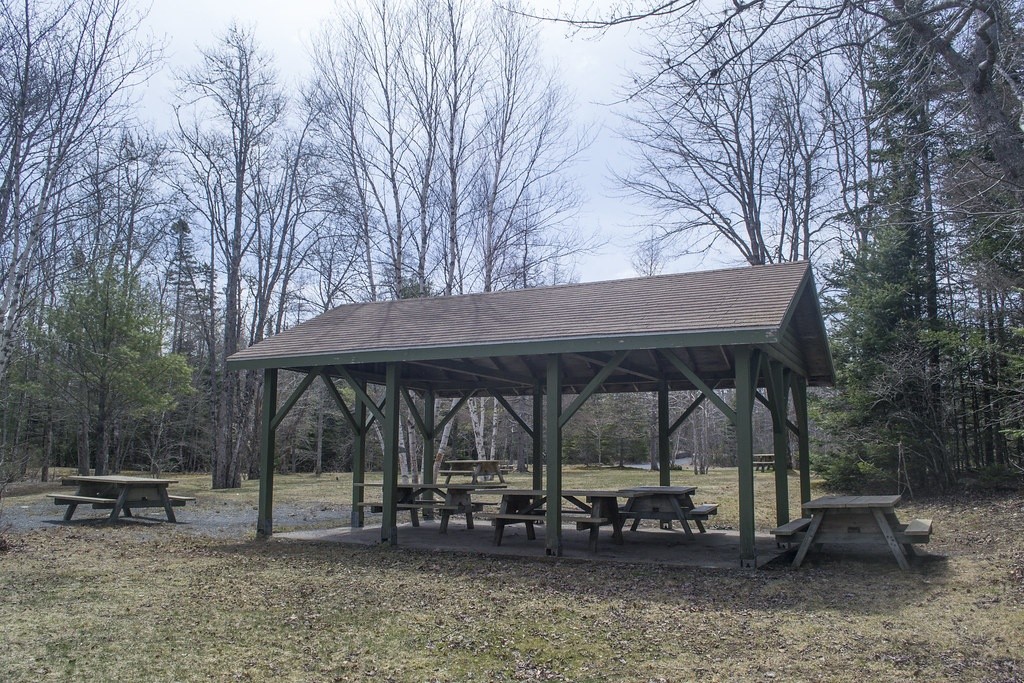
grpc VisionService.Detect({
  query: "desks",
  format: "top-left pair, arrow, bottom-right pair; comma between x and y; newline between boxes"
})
612,487 -> 717,537
467,489 -> 623,554
353,483 -> 507,533
776,495 -> 932,569
54,476 -> 187,524
752,454 -> 775,472
445,460 -> 505,484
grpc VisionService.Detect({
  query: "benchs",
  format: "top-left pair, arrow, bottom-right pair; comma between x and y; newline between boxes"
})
357,500 -> 497,510
753,462 -> 775,465
688,504 -> 719,514
439,470 -> 474,476
45,494 -> 116,504
770,518 -> 812,535
475,508 -> 607,523
168,495 -> 196,501
904,520 -> 933,535
